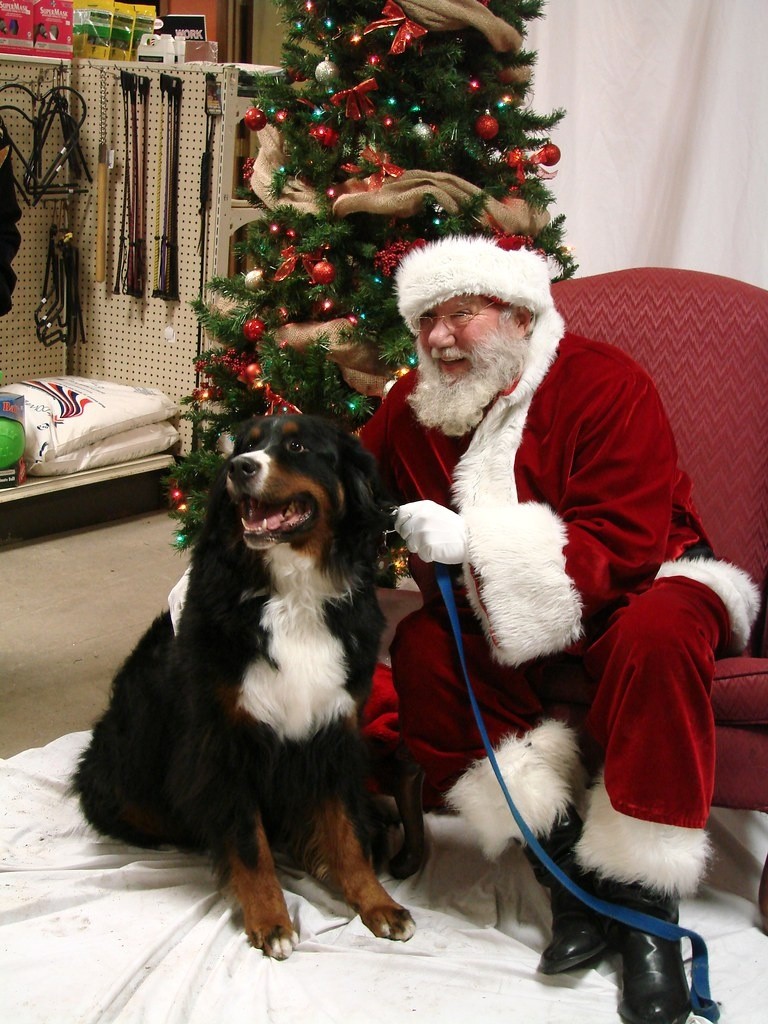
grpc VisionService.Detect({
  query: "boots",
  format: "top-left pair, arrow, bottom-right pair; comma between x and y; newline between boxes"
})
614,879 -> 692,1024
514,805 -> 615,975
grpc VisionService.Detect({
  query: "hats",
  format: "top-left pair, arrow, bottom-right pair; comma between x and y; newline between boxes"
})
393,233 -> 563,321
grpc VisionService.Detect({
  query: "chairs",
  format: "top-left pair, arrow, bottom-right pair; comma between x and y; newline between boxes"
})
551,267 -> 768,935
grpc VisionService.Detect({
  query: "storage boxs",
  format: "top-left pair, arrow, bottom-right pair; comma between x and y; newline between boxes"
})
0,392 -> 26,489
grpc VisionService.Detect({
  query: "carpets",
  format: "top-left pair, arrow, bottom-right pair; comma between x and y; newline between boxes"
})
0,730 -> 768,1024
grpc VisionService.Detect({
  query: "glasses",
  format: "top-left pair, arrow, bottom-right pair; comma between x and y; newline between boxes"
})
409,302 -> 496,332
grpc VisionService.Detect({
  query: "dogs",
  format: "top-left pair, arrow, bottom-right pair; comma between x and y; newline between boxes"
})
77,415 -> 418,958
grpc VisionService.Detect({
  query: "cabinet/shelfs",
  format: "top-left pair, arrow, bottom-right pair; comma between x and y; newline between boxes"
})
0,0 -> 279,547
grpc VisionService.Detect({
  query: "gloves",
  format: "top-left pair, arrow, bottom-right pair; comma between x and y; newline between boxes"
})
390,500 -> 469,565
167,565 -> 192,635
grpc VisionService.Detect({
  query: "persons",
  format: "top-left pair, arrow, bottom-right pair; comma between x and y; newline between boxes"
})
347,234 -> 761,1024
